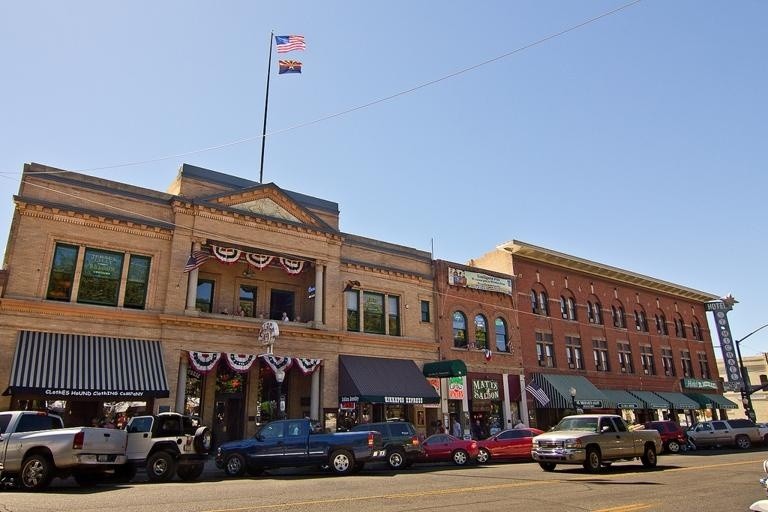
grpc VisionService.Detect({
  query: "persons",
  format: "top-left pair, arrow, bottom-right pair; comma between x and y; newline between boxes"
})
433,420 -> 441,435
452,417 -> 461,439
513,419 -> 525,429
472,420 -> 482,438
438,424 -> 444,433
220,305 -> 302,322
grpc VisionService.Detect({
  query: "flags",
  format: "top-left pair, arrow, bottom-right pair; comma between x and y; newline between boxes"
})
183,251 -> 209,273
523,380 -> 550,406
277,60 -> 302,74
275,35 -> 304,53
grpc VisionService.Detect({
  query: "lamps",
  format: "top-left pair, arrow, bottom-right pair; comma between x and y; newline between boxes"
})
243,263 -> 256,278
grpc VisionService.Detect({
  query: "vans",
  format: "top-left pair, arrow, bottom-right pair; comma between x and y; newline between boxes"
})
684,417 -> 765,450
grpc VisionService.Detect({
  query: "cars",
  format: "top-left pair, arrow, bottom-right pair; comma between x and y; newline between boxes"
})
756,421 -> 768,448
747,458 -> 768,512
417,427 -> 481,468
639,421 -> 689,453
477,426 -> 546,463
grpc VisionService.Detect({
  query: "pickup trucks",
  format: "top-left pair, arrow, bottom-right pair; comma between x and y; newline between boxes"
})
213,418 -> 389,478
0,408 -> 133,494
529,413 -> 667,475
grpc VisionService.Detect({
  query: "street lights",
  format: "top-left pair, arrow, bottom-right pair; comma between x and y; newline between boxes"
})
273,368 -> 286,419
743,395 -> 752,419
568,386 -> 578,412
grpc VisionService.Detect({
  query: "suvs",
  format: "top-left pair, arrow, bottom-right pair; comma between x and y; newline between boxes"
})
116,406 -> 216,483
346,419 -> 428,474
